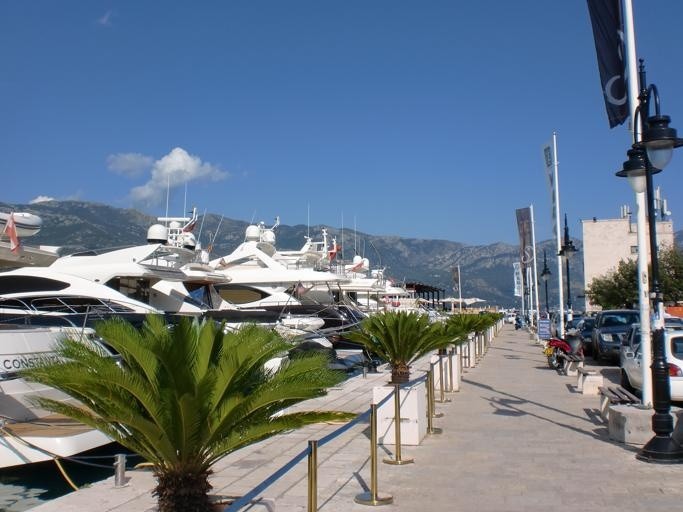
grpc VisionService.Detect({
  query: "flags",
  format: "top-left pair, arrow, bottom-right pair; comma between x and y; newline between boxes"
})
510,261 -> 522,297
514,206 -> 534,269
451,266 -> 459,283
325,245 -> 341,261
2,212 -> 21,256
539,140 -> 560,251
585,0 -> 631,130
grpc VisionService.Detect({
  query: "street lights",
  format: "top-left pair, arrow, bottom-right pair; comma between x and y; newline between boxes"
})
613,56 -> 682,463
539,249 -> 553,313
556,212 -> 578,323
524,275 -> 542,307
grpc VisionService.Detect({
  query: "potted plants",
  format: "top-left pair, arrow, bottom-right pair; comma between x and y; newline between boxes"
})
339,310 -> 505,445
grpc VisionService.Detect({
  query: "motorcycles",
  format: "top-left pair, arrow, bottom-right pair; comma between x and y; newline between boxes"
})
513,316 -> 526,330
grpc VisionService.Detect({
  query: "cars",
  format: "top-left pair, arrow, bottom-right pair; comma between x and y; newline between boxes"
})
479,309 -> 519,325
519,307 -> 682,401
